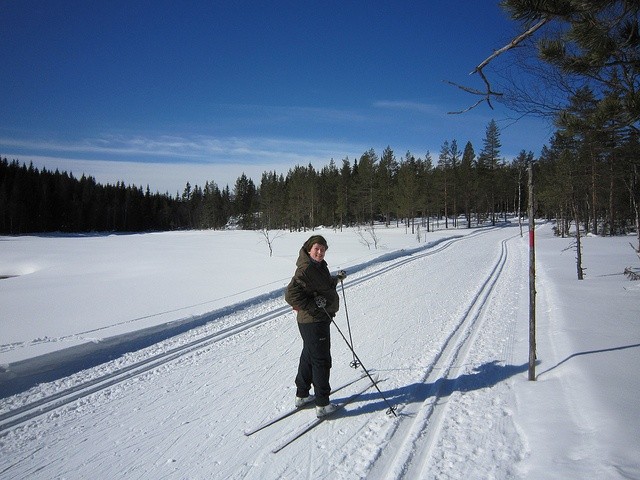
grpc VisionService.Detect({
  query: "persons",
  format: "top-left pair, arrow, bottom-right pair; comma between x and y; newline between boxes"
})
284,235 -> 347,418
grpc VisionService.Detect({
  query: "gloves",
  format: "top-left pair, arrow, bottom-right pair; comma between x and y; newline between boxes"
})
314,296 -> 327,308
337,270 -> 346,279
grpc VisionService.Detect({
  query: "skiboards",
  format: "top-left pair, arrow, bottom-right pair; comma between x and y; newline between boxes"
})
244,369 -> 379,453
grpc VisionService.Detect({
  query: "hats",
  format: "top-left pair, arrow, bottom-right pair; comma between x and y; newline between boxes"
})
304,236 -> 328,252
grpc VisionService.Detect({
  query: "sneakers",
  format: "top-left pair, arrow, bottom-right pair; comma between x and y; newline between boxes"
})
295,395 -> 316,406
316,403 -> 336,416
